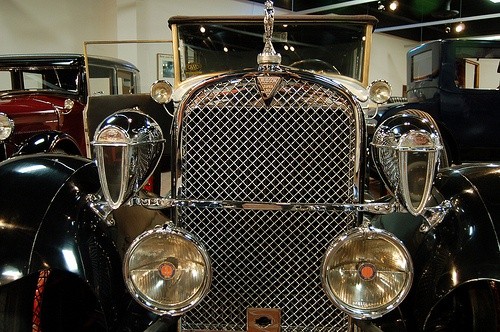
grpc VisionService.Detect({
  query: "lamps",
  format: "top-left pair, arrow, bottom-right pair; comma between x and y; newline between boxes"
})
389,0 -> 400,11
454,0 -> 465,33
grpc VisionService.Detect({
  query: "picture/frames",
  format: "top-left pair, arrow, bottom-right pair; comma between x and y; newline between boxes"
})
157,53 -> 175,85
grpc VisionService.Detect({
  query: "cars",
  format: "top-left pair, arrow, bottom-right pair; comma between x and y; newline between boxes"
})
1,1 -> 500,331
